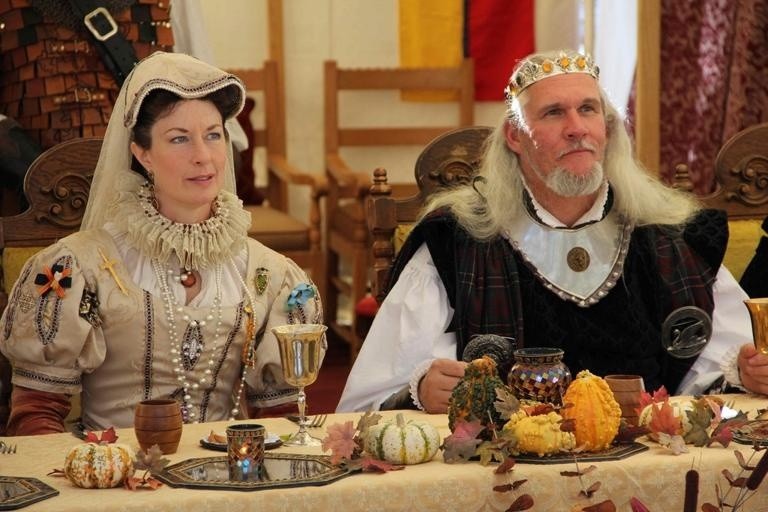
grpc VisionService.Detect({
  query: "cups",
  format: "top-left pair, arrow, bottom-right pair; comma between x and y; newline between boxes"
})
604,372 -> 649,436
746,300 -> 767,354
225,425 -> 265,479
136,401 -> 181,455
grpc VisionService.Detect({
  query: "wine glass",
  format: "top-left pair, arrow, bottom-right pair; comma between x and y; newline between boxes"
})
273,326 -> 327,447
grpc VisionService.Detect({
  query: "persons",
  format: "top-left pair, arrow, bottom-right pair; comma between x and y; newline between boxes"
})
1,49 -> 324,438
333,47 -> 768,416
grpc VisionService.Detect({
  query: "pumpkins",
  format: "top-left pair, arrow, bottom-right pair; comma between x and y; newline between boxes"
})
363,412 -> 440,465
64,438 -> 131,489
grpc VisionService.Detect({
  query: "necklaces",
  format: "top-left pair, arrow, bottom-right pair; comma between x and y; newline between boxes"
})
151,251 -> 257,424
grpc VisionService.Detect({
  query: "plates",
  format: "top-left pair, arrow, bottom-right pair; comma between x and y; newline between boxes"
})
640,399 -> 739,434
